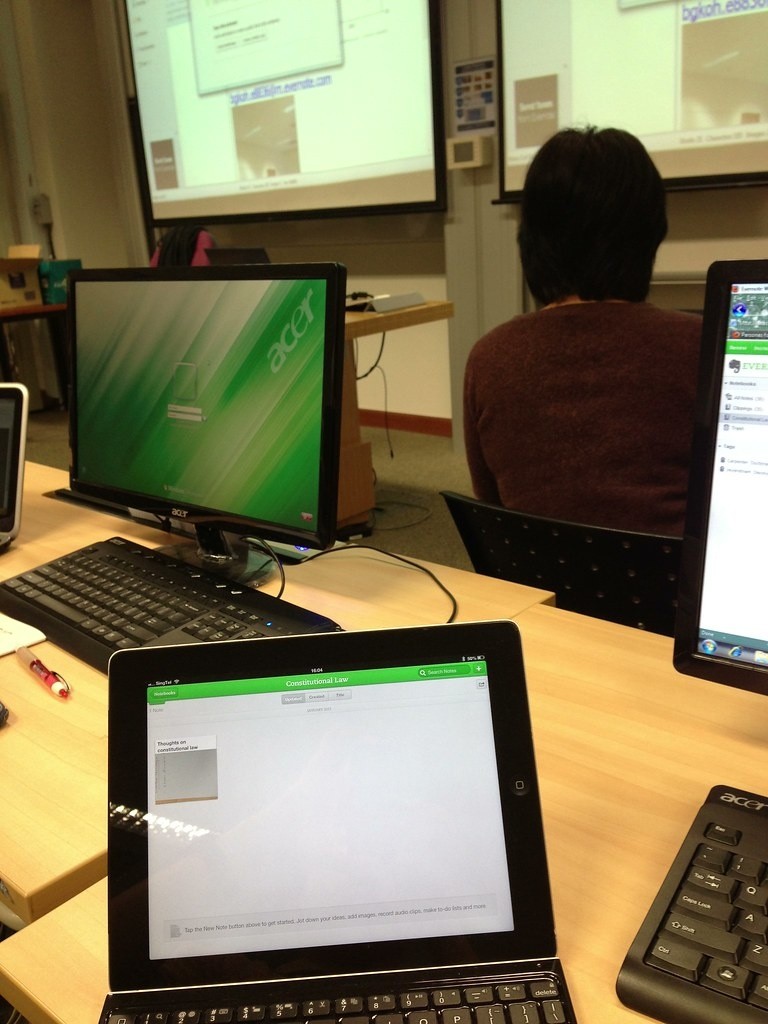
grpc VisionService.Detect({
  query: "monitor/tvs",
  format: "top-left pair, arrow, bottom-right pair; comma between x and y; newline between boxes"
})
65,262 -> 348,588
672,258 -> 768,700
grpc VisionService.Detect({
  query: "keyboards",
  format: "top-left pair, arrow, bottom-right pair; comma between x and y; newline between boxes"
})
616,785 -> 768,1024
0,535 -> 348,677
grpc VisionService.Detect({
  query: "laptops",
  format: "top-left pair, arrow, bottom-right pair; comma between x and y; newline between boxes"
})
99,619 -> 579,1024
0,382 -> 29,552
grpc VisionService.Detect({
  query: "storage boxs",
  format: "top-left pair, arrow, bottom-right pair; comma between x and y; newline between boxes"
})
38,258 -> 83,305
2,244 -> 43,306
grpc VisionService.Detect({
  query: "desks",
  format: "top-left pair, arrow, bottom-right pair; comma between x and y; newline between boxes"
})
0,459 -> 559,927
335,293 -> 455,546
0,304 -> 70,415
0,601 -> 768,1024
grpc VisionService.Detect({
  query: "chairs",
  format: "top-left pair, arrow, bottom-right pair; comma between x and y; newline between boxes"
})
439,489 -> 684,637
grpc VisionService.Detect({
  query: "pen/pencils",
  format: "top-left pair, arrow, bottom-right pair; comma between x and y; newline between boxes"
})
6,639 -> 70,699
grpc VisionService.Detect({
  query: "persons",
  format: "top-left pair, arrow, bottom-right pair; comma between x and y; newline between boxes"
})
461,126 -> 702,636
